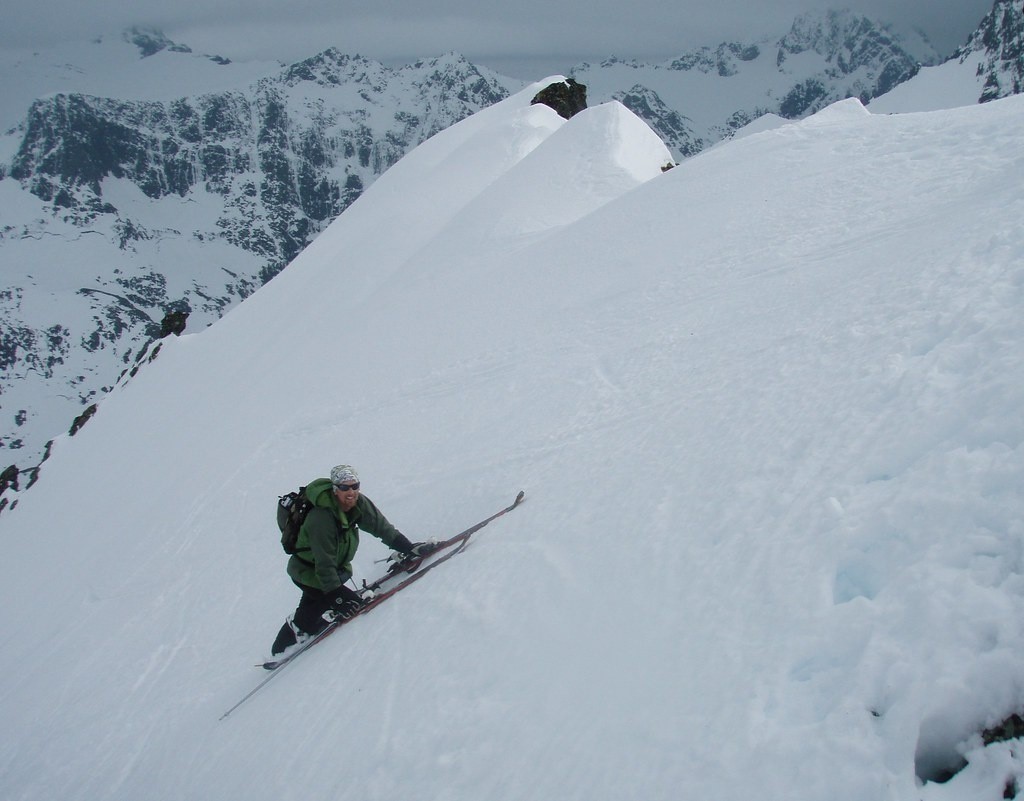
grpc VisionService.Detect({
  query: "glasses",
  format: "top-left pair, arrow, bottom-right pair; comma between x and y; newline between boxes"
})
332,482 -> 360,491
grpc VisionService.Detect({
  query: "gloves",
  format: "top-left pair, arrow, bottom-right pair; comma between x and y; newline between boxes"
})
405,542 -> 434,557
327,586 -> 363,613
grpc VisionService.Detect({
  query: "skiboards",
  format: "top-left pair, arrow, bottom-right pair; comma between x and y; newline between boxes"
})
259,487 -> 526,672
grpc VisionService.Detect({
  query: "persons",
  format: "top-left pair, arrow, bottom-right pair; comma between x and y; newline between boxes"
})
271,464 -> 427,656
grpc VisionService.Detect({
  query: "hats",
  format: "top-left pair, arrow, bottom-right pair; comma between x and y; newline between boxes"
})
331,464 -> 359,491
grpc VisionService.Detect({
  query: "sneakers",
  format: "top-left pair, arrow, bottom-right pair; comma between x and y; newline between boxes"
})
321,610 -> 337,623
286,613 -> 309,645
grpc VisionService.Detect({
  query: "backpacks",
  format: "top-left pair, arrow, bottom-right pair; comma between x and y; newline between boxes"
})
277,486 -> 314,554
